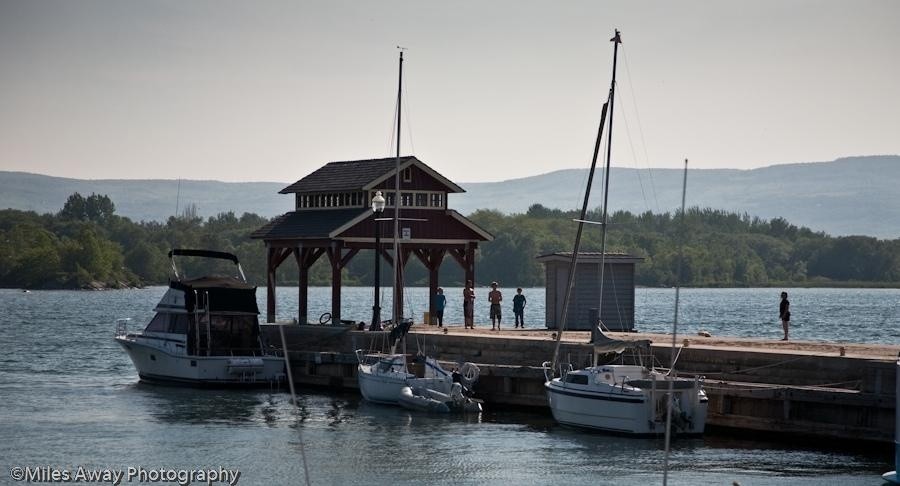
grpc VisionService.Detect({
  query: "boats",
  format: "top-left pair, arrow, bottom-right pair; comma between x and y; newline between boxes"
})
112,246 -> 285,394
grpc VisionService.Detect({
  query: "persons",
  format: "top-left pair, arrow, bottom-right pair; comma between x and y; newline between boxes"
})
512,288 -> 527,328
433,287 -> 447,328
463,280 -> 476,329
488,282 -> 503,330
779,291 -> 791,340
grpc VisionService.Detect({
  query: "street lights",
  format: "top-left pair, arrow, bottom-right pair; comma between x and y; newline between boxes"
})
368,190 -> 388,331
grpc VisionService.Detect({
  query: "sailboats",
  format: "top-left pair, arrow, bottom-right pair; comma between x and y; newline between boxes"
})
356,44 -> 486,417
541,30 -> 710,436
